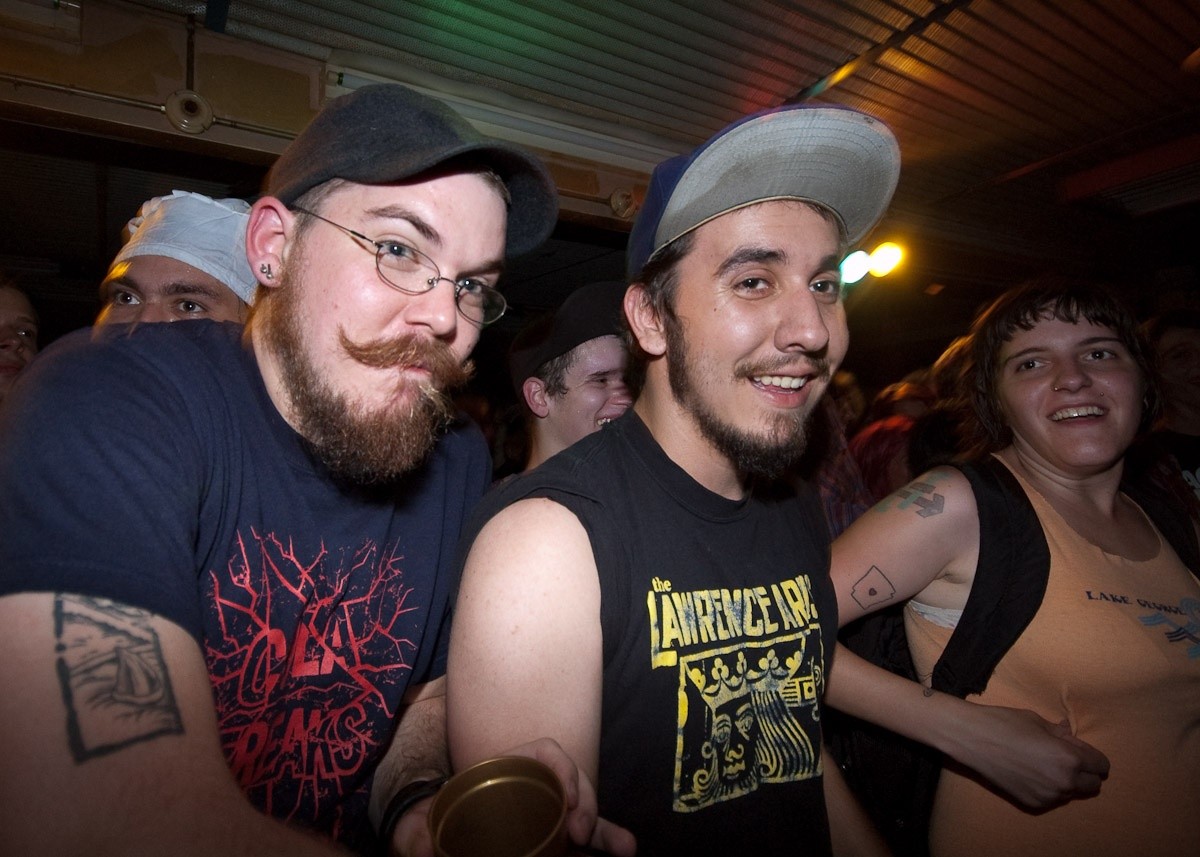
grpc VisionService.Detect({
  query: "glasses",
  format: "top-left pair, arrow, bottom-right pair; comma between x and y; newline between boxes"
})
284,203 -> 507,323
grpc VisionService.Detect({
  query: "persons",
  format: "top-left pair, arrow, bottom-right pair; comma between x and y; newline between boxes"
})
0,271 -> 38,388
1120,306 -> 1200,578
509,281 -> 635,475
442,101 -> 901,857
0,84 -> 638,857
830,274 -> 1200,857
95,189 -> 260,324
826,334 -> 972,782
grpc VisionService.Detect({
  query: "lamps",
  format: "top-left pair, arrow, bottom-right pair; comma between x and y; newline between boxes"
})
327,71 -> 671,164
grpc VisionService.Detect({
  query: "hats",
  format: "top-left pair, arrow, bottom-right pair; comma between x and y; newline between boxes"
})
628,106 -> 901,279
106,189 -> 261,307
501,281 -> 631,388
259,81 -> 557,259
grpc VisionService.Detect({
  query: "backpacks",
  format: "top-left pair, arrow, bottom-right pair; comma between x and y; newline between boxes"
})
820,448 -> 1190,855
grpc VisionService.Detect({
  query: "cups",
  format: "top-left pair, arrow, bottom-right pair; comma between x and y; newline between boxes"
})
427,755 -> 568,857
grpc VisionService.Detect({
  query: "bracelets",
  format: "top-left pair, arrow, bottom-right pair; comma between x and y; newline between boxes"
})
377,780 -> 442,853
